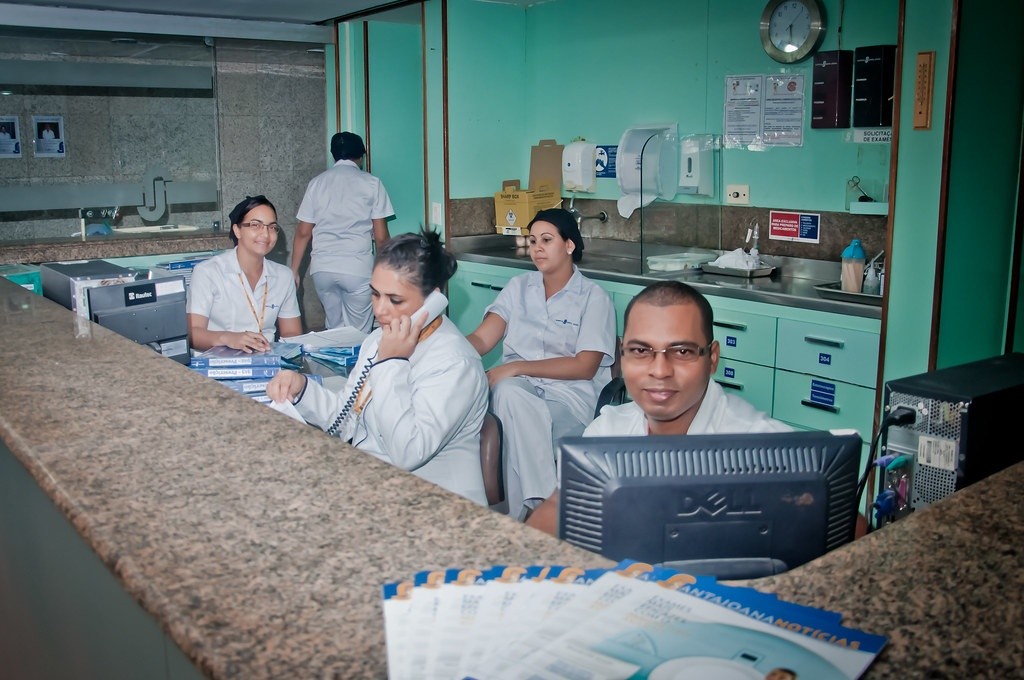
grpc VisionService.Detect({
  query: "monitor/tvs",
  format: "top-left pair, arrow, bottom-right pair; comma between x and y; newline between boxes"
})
557,429 -> 863,581
87,275 -> 191,365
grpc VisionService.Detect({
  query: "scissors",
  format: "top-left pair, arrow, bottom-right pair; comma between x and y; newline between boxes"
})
848,176 -> 868,196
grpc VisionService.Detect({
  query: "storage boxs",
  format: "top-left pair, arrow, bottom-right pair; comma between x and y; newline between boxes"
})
495,139 -> 564,236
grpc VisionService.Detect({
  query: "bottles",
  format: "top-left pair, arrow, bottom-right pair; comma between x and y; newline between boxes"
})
211,212 -> 221,232
864,268 -> 880,294
840,239 -> 866,294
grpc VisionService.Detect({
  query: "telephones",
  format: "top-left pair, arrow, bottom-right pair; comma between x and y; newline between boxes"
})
400,290 -> 450,332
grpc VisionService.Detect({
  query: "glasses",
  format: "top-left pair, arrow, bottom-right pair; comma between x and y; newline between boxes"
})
618,339 -> 715,363
239,219 -> 280,235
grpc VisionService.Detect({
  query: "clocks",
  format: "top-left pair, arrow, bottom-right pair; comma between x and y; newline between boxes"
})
758,0 -> 827,64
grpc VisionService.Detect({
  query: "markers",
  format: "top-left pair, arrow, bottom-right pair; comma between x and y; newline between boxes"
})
160,226 -> 179,229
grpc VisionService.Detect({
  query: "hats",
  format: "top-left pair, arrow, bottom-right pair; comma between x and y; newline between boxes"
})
331,132 -> 367,158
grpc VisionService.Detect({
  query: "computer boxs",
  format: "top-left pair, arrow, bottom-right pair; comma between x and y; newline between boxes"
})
41,259 -> 139,321
875,352 -> 1024,530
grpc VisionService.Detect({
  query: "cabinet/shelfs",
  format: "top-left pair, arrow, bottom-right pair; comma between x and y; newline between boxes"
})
444,260 -> 884,530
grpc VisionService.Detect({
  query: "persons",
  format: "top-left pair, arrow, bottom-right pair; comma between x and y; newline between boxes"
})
765,668 -> 796,680
525,280 -> 867,539
266,223 -> 489,508
466,208 -> 617,522
186,194 -> 302,354
42,124 -> 54,139
291,132 -> 396,334
0,125 -> 10,138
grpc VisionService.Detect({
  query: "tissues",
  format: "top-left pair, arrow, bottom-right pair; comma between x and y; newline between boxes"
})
613,127 -> 678,219
